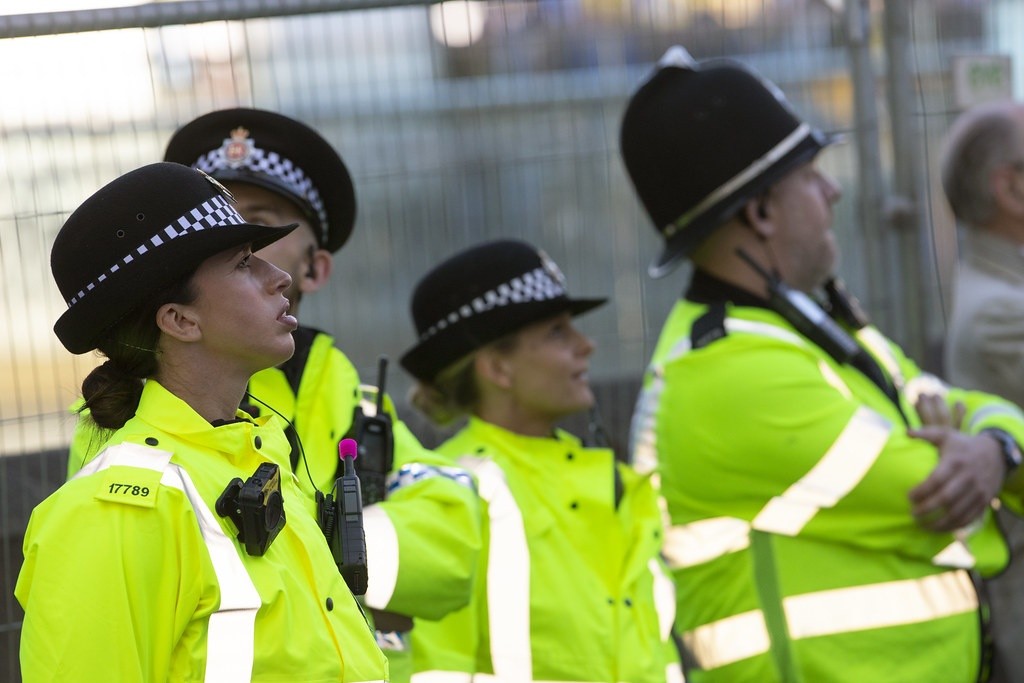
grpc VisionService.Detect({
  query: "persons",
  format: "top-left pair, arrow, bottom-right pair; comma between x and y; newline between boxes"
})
943,102 -> 1024,683
617,55 -> 1024,683
67,107 -> 483,683
15,164 -> 393,683
396,242 -> 634,682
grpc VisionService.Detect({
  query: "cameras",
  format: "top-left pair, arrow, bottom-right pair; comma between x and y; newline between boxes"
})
215,462 -> 286,557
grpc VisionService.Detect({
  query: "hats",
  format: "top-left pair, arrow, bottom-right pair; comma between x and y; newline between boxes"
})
164,109 -> 356,254
397,240 -> 608,381
619,57 -> 847,277
49,162 -> 300,356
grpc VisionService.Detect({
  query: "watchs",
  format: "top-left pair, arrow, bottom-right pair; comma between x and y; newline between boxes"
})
989,430 -> 1023,476
